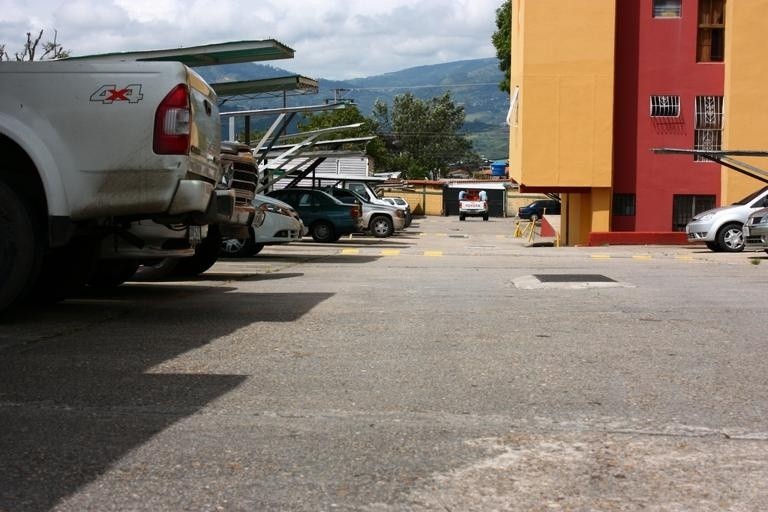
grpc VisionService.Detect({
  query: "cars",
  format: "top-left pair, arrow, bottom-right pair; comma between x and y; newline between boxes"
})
317,187 -> 406,240
518,200 -> 562,223
742,206 -> 768,255
213,188 -> 304,258
686,184 -> 768,252
262,189 -> 359,242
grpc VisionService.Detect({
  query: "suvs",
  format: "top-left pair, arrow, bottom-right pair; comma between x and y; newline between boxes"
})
378,195 -> 411,223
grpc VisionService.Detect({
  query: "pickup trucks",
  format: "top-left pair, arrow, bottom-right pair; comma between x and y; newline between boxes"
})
60,135 -> 259,292
459,188 -> 488,221
0,62 -> 222,323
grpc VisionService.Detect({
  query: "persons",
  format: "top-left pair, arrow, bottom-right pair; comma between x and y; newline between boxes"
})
478,189 -> 488,201
458,189 -> 469,201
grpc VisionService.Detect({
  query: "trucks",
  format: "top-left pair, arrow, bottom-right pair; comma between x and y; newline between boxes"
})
260,152 -> 391,206
